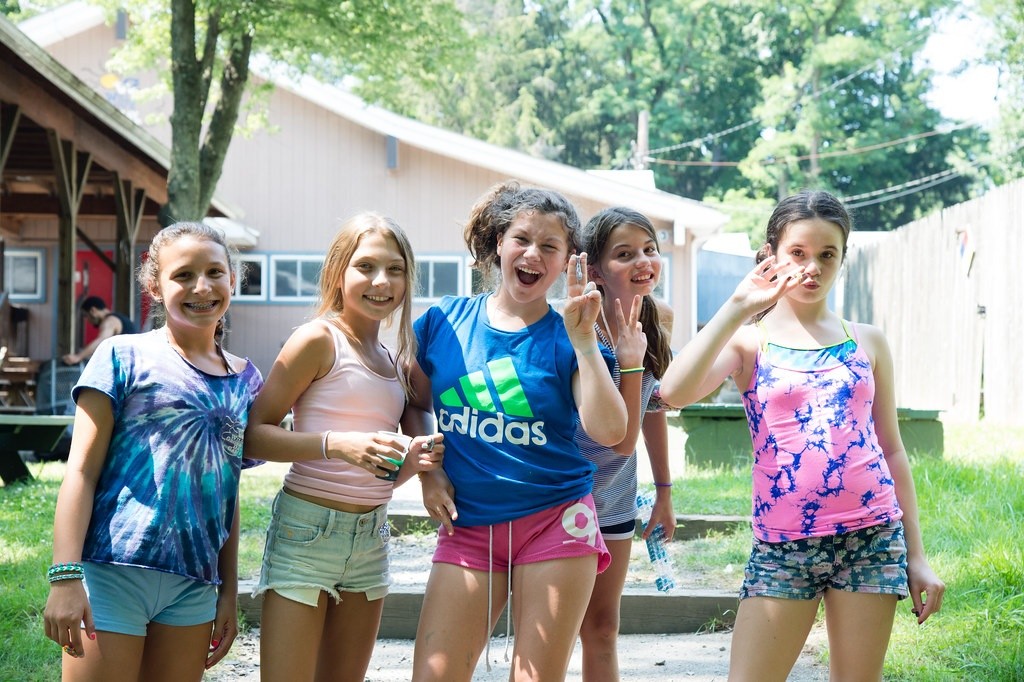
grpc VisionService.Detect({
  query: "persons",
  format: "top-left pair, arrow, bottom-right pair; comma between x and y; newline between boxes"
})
44,220 -> 266,682
659,190 -> 947,681
62,296 -> 136,366
243,214 -> 444,682
400,178 -> 630,682
579,206 -> 678,681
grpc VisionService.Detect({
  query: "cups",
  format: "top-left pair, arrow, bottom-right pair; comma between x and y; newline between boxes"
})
374,430 -> 412,482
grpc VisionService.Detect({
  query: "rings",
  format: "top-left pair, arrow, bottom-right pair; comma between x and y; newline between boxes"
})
61,642 -> 76,654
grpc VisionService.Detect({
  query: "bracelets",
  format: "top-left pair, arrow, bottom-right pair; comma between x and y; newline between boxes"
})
323,430 -> 332,460
47,562 -> 84,582
653,482 -> 673,486
618,367 -> 645,374
